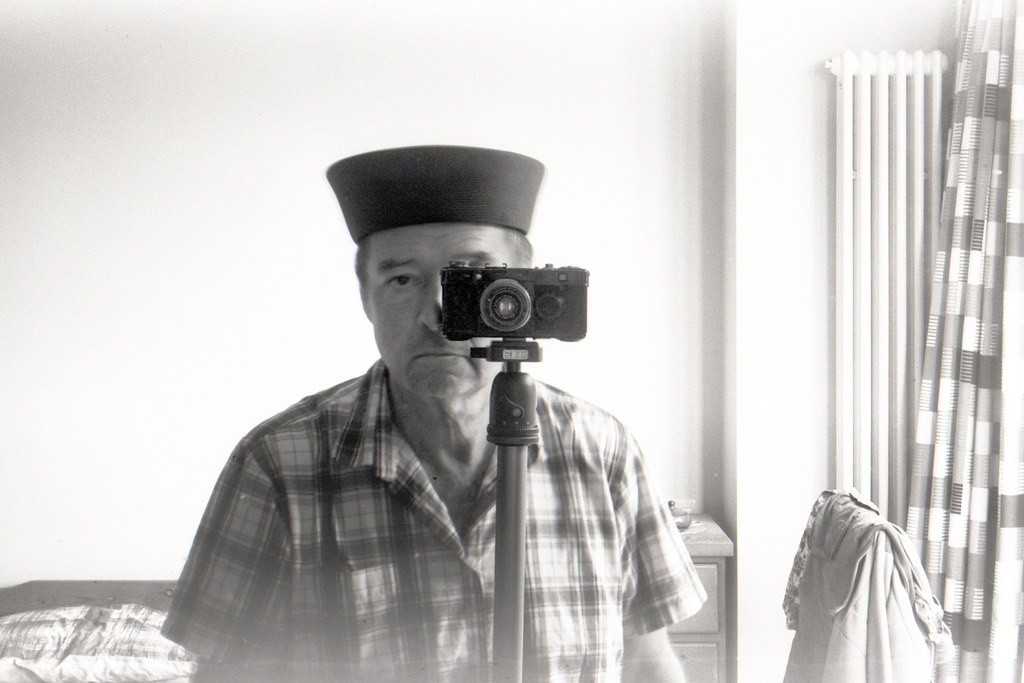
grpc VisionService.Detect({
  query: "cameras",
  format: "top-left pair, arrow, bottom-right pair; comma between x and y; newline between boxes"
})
440,260 -> 590,342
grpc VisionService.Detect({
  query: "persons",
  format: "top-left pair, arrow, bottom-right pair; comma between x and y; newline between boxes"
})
160,145 -> 711,683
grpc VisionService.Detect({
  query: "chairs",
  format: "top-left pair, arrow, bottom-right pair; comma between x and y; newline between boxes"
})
782,492 -> 955,683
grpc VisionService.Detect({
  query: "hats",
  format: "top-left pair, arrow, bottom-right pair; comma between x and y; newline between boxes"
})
327,145 -> 546,243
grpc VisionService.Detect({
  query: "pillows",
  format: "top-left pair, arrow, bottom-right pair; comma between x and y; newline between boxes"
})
15,603 -> 198,683
0,605 -> 94,683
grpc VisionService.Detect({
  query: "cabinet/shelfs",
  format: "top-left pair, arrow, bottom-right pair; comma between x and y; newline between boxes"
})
666,513 -> 734,683
